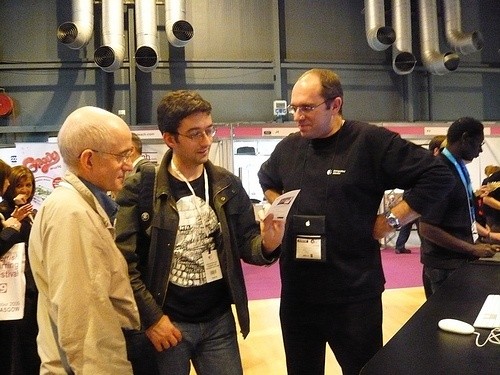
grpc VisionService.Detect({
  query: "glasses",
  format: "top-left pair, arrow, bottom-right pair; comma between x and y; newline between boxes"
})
473,137 -> 487,146
288,95 -> 339,113
77,147 -> 135,162
167,127 -> 216,141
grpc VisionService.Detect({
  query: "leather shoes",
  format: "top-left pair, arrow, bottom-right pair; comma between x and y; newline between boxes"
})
396,246 -> 411,254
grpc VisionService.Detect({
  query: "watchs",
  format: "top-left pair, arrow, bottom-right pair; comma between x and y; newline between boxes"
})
383,208 -> 403,232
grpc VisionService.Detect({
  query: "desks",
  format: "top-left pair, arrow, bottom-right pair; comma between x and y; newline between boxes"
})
357,230 -> 500,375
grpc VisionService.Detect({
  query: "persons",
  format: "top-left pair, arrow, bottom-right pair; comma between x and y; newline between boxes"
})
26,104 -> 152,374
417,115 -> 497,302
5,164 -> 43,375
113,87 -> 286,374
391,133 -> 500,261
122,128 -> 156,181
1,156 -> 36,375
256,68 -> 456,374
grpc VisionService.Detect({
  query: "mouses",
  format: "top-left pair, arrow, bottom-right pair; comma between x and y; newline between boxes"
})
438,318 -> 475,334
